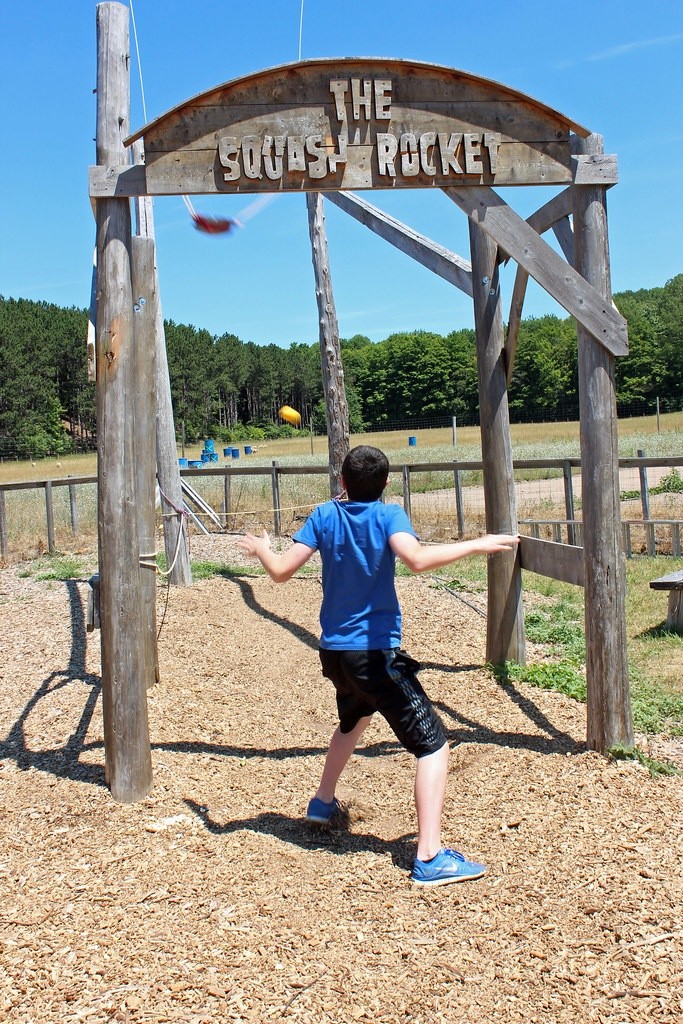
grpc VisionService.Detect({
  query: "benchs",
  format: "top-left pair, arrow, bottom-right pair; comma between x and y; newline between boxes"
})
650,569 -> 683,628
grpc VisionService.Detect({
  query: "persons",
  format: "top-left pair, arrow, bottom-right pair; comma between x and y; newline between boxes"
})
237,445 -> 522,886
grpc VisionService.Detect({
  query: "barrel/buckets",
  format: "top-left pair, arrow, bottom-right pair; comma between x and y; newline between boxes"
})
188,461 -> 203,469
223,449 -> 231,457
201,439 -> 218,463
178,457 -> 188,469
409,437 -> 416,446
244,446 -> 251,455
228,447 -> 234,454
231,449 -> 239,458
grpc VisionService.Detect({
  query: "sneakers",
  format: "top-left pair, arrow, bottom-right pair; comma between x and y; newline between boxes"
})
306,796 -> 342,824
413,849 -> 487,887
